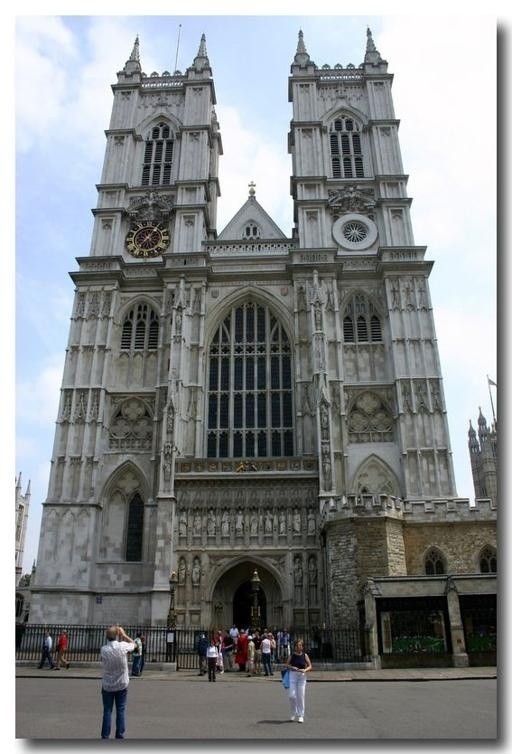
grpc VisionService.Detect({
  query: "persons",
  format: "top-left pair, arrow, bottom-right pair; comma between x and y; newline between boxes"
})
284,638 -> 313,722
55,628 -> 71,670
132,631 -> 144,676
100,624 -> 138,739
197,623 -> 292,682
139,635 -> 147,672
36,631 -> 57,669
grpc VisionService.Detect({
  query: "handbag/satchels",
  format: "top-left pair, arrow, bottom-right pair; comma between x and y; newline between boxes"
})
282,670 -> 290,689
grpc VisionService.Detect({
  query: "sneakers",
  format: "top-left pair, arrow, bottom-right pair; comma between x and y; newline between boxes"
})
209,680 -> 215,682
37,664 -> 70,670
264,673 -> 273,676
290,713 -> 296,721
298,717 -> 303,723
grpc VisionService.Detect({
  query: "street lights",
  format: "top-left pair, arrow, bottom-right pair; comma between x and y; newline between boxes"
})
247,568 -> 263,628
166,570 -> 179,629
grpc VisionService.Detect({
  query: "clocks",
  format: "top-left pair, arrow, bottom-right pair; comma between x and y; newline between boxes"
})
124,221 -> 170,259
